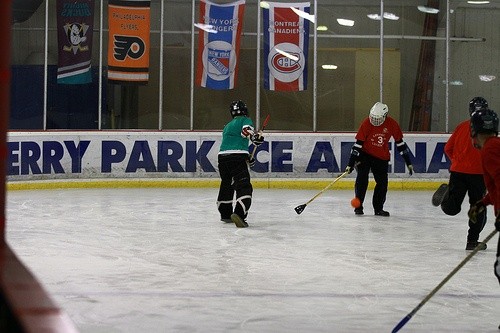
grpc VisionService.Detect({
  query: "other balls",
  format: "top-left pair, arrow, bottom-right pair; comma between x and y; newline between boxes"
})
351,198 -> 360,209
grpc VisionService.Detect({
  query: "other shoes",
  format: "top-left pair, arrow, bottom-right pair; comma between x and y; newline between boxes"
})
221,217 -> 232,223
375,209 -> 390,216
230,212 -> 248,227
466,242 -> 487,250
432,183 -> 445,207
355,210 -> 364,214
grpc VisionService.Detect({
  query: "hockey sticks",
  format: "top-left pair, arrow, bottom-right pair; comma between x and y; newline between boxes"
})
391,228 -> 500,332
231,114 -> 272,191
294,159 -> 363,216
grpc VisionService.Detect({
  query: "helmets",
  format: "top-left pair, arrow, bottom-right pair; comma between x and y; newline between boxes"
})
469,97 -> 489,117
369,102 -> 389,126
230,99 -> 249,118
469,108 -> 499,137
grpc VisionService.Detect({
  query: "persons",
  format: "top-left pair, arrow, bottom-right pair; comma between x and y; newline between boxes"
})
432,97 -> 490,251
216,100 -> 265,227
345,103 -> 413,217
470,109 -> 500,330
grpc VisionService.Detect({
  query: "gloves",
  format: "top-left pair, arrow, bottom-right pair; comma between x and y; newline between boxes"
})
345,165 -> 354,174
408,165 -> 415,176
248,154 -> 256,167
252,134 -> 265,147
